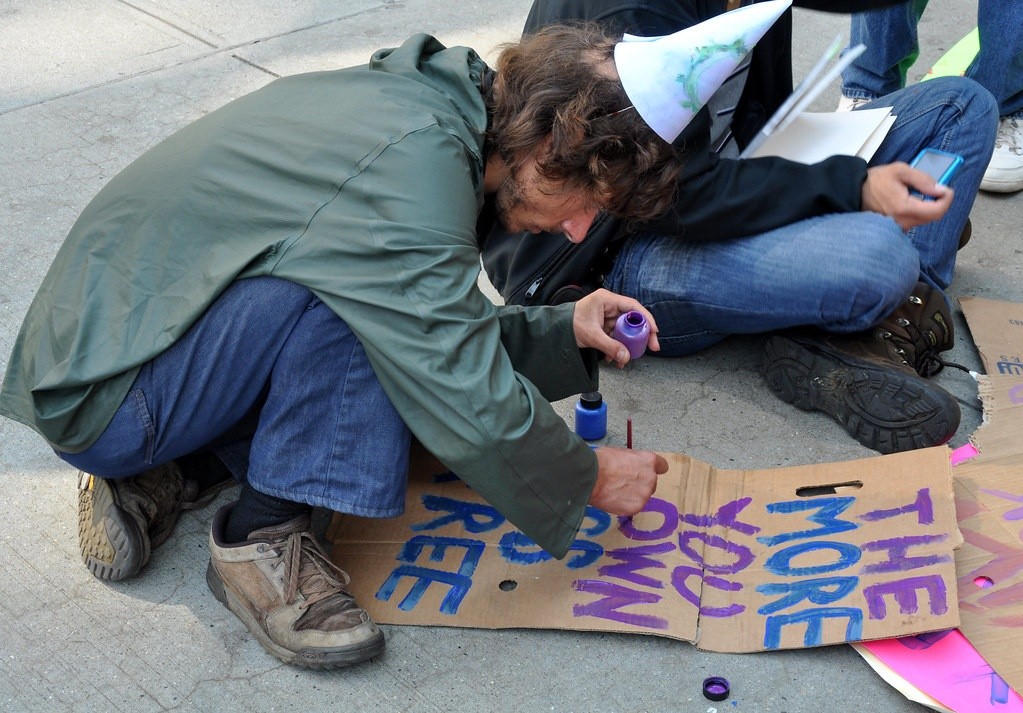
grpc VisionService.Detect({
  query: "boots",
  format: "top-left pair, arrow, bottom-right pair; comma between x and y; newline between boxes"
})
756,278 -> 959,457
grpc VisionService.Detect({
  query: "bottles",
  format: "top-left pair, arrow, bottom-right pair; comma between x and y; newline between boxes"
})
573,393 -> 607,439
615,309 -> 650,361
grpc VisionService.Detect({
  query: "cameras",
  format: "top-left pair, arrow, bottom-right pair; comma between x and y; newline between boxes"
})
906,147 -> 964,201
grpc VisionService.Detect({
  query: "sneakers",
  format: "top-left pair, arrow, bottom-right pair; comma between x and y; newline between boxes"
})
205,502 -> 384,669
77,467 -> 193,585
980,116 -> 1022,193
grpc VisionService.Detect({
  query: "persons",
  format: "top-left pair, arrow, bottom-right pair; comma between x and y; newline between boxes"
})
481,0 -> 998,459
835,0 -> 1022,192
0,17 -> 683,666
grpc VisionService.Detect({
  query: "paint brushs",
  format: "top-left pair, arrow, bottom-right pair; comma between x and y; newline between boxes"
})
627,419 -> 633,522
